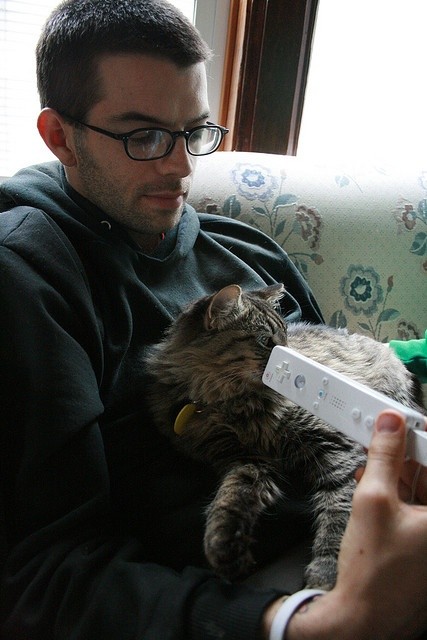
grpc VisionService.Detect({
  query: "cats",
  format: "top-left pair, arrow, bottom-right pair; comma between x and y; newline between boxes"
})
139,280 -> 427,594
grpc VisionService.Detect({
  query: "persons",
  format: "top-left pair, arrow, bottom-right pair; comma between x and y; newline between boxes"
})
1,0 -> 427,639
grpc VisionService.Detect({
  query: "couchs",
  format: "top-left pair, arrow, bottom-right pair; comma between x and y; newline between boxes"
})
186,151 -> 427,345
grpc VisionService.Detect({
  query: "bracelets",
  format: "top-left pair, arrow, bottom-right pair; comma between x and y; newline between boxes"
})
271,588 -> 328,639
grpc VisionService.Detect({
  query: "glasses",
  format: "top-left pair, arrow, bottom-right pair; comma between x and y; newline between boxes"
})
68,115 -> 232,162
